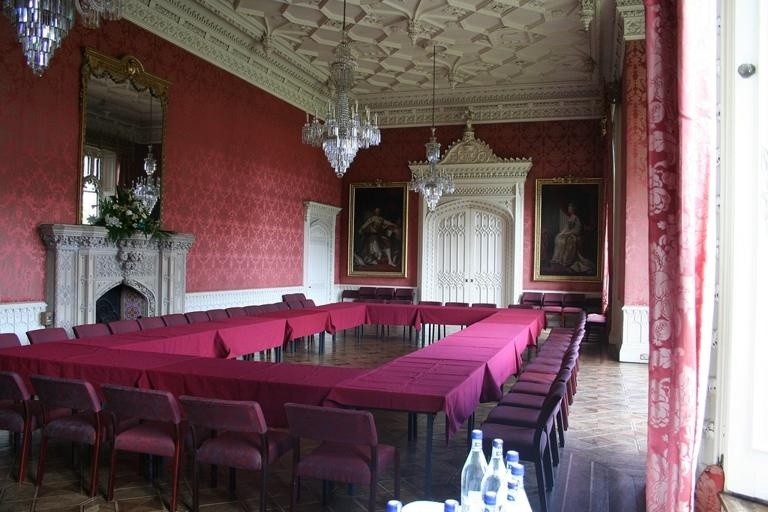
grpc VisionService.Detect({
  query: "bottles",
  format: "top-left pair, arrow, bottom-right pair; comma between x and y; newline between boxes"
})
388,430 -> 534,511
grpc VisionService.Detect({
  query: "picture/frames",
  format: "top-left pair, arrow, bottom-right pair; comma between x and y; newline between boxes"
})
533,176 -> 607,283
347,179 -> 409,278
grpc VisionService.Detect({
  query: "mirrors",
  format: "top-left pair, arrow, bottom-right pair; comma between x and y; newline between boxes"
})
75,48 -> 174,225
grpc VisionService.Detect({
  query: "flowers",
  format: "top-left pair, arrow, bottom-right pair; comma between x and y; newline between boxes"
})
84,195 -> 119,226
105,191 -> 179,246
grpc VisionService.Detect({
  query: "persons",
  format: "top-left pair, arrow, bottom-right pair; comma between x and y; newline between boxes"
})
358,206 -> 402,268
547,202 -> 594,274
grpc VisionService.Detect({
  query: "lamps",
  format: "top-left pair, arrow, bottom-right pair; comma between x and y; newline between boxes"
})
410,45 -> 455,212
128,94 -> 161,216
3,0 -> 122,78
301,0 -> 382,180
